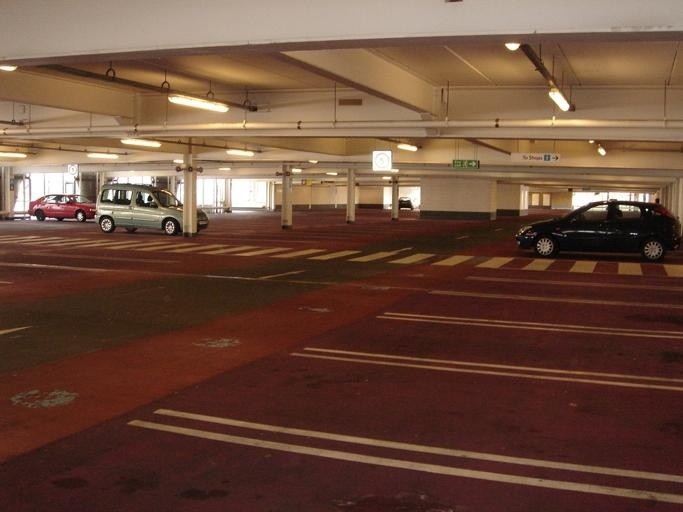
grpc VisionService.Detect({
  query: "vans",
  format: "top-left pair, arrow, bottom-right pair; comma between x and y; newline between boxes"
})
93,184 -> 209,236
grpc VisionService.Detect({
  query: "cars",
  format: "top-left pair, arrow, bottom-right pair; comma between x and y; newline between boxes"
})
398,197 -> 413,210
26,194 -> 95,222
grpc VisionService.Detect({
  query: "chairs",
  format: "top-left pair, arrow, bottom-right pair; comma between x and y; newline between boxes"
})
136,194 -> 146,207
147,195 -> 153,201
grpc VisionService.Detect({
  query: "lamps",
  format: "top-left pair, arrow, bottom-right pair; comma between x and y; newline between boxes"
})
504,43 -> 608,159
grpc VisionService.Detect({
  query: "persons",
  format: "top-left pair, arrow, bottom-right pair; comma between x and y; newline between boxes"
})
653,198 -> 663,215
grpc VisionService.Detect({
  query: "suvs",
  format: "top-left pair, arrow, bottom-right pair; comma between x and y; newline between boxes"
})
514,198 -> 680,261
